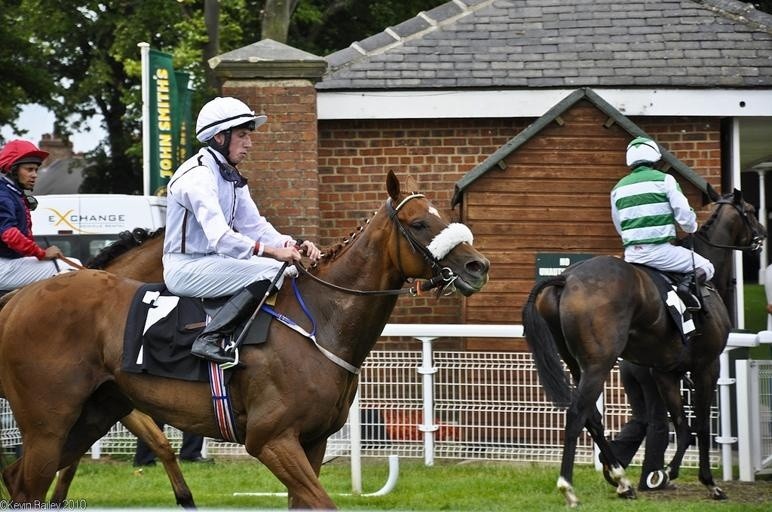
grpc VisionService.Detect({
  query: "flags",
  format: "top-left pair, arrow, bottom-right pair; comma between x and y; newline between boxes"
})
140,43 -> 200,197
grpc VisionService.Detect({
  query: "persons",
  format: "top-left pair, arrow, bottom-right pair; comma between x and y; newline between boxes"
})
0,139 -> 84,292
132,414 -> 219,471
160,94 -> 325,365
598,358 -> 678,492
607,134 -> 717,317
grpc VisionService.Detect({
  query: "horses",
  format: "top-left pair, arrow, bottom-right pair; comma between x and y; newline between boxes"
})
1,168 -> 490,510
521,181 -> 770,509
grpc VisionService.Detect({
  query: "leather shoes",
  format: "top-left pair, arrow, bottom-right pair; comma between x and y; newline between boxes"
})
180,455 -> 215,465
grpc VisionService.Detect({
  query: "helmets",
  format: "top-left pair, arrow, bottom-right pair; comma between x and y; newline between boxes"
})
626,135 -> 662,166
196,97 -> 267,142
0,140 -> 49,173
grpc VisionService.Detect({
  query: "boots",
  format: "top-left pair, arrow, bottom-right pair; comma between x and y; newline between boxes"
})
676,268 -> 706,313
190,286 -> 261,368
